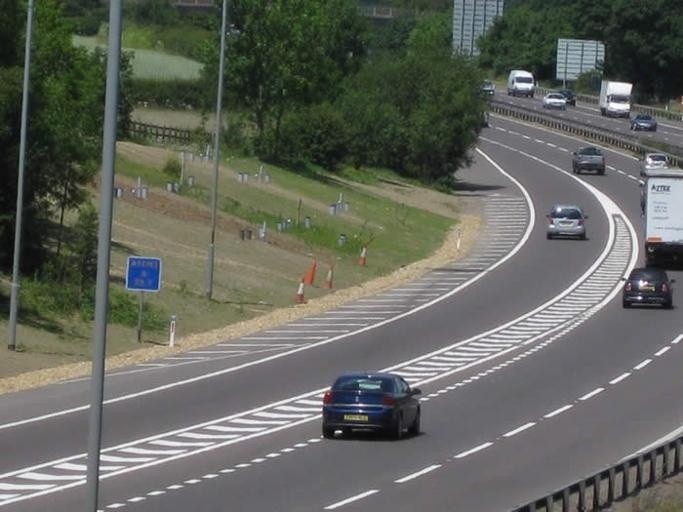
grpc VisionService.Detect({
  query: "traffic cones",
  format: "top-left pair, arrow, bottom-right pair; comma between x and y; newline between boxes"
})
356,245 -> 369,265
305,260 -> 319,286
294,276 -> 306,304
321,264 -> 336,289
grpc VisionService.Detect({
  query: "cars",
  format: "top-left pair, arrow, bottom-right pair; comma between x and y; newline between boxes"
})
481,79 -> 494,96
559,90 -> 577,106
321,370 -> 422,438
543,92 -> 567,112
618,267 -> 677,308
639,153 -> 669,177
545,204 -> 587,239
630,114 -> 657,131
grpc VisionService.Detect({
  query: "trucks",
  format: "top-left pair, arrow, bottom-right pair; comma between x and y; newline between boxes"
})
507,69 -> 535,99
640,167 -> 682,269
571,146 -> 607,174
599,80 -> 633,118
